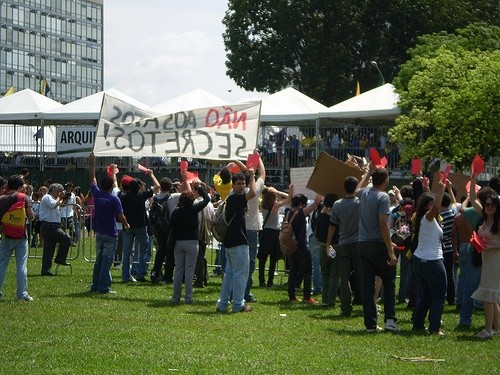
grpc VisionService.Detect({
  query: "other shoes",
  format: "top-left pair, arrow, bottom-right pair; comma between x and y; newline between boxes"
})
245,294 -> 257,303
40,271 -> 57,276
109,290 -> 118,293
290,296 -> 317,304
131,275 -> 208,287
414,328 -> 445,336
474,330 -> 500,339
259,282 -> 273,286
172,298 -> 193,304
233,305 -> 251,312
366,319 -> 400,333
54,260 -> 71,266
17,294 -> 34,301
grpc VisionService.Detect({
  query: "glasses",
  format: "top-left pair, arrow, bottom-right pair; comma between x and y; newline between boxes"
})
485,202 -> 497,207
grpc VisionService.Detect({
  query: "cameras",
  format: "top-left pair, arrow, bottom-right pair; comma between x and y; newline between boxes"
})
65,192 -> 71,196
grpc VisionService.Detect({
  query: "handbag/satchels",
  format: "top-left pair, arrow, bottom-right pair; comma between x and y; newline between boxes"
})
1,192 -> 26,238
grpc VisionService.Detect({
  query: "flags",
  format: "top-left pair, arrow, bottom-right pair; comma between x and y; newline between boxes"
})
93,93 -> 262,161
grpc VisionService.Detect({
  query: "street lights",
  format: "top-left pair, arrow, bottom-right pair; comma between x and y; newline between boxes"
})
371,61 -> 385,87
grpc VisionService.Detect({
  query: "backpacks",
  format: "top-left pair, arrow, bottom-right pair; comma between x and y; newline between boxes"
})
279,210 -> 298,257
210,193 -> 237,242
148,192 -> 172,232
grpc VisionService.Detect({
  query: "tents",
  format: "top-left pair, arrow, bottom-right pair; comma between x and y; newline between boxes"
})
0,83 -> 401,181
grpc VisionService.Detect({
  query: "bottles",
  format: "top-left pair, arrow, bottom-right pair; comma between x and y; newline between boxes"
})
329,246 -> 336,258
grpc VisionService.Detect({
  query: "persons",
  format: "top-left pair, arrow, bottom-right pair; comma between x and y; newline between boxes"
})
0,167 -> 97,248
257,122 -> 399,167
99,156 -> 206,174
0,175 -> 34,301
0,150 -> 25,166
87,149 -> 500,339
39,183 -> 71,275
